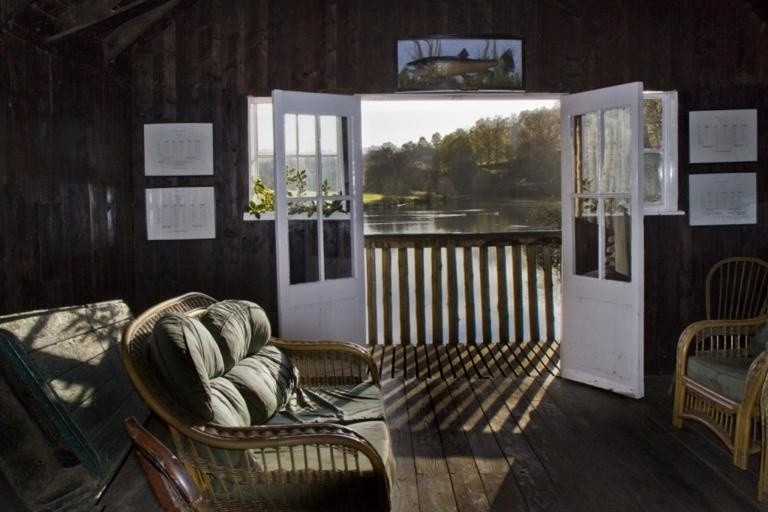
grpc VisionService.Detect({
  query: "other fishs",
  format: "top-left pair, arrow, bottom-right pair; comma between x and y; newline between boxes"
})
405,47 -> 516,78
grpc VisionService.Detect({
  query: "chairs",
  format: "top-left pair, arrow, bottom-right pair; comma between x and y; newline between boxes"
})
668,256 -> 767,472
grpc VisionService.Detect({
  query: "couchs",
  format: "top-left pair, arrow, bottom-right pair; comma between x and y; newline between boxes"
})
120,290 -> 396,512
